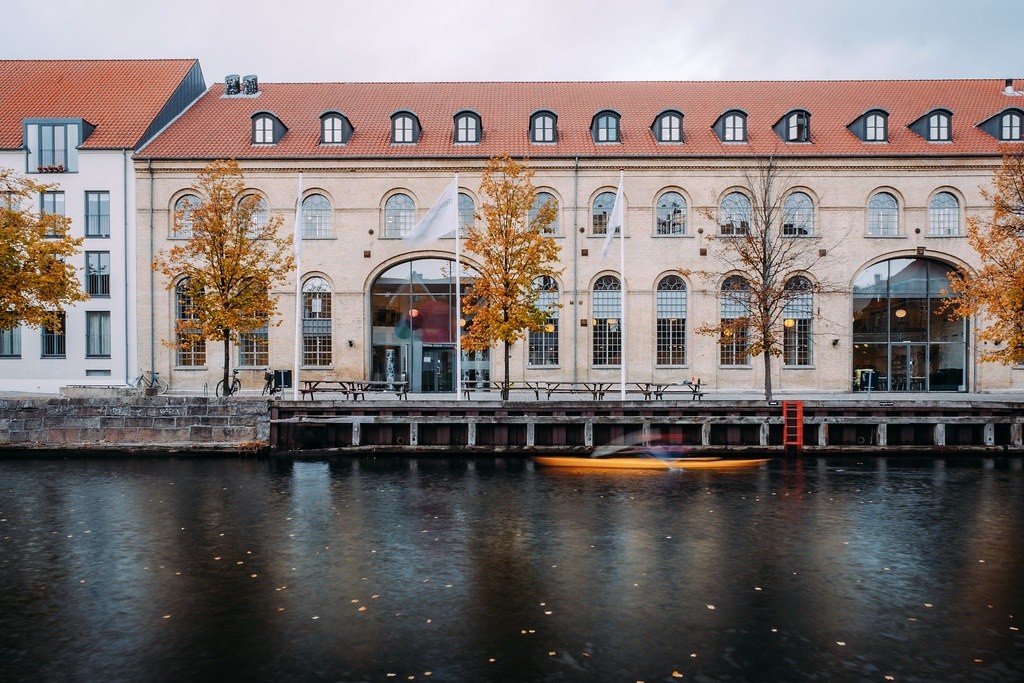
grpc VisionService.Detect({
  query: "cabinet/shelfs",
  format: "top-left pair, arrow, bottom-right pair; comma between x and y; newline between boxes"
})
892,360 -> 907,391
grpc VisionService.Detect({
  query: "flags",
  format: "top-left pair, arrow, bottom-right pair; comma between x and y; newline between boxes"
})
603,176 -> 623,263
293,174 -> 302,263
403,178 -> 460,248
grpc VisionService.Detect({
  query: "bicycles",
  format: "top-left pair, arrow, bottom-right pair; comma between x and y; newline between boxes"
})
131,367 -> 169,395
261,366 -> 283,398
216,368 -> 241,398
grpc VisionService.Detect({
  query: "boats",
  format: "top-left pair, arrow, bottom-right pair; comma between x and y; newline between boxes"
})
528,452 -> 772,470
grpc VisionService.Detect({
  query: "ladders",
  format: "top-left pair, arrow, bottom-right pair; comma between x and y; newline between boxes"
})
783,400 -> 803,452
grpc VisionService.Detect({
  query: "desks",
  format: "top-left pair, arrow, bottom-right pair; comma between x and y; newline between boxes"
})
911,377 -> 926,391
546,381 -> 597,400
461,381 -> 502,400
503,380 -> 546,401
651,382 -> 710,400
352,380 -> 408,400
300,380 -> 353,400
878,377 -> 894,391
597,382 -> 652,400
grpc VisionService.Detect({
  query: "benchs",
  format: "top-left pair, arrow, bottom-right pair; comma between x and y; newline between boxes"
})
298,389 -> 350,392
66,384 -> 127,389
503,387 -> 546,390
655,391 -> 710,395
355,388 -> 413,391
309,387 -> 348,390
455,387 -> 501,390
651,391 -> 702,393
546,389 -> 595,392
351,391 -> 404,394
597,390 -> 650,393
546,391 -> 597,393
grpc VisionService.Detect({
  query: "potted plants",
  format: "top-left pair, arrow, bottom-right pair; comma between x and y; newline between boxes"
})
37,165 -> 42,171
43,166 -> 47,170
58,160 -> 65,171
54,163 -> 57,170
48,161 -> 53,170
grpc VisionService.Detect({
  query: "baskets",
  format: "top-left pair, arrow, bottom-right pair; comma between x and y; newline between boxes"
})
264,372 -> 275,381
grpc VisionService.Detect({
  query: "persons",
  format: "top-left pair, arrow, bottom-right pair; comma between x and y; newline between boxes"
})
463,372 -> 470,398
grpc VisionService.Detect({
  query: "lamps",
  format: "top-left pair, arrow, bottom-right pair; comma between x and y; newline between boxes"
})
785,319 -> 795,329
545,324 -> 554,333
896,308 -> 907,319
607,318 -> 616,325
460,319 -> 466,327
408,300 -> 418,317
724,328 -> 734,338
